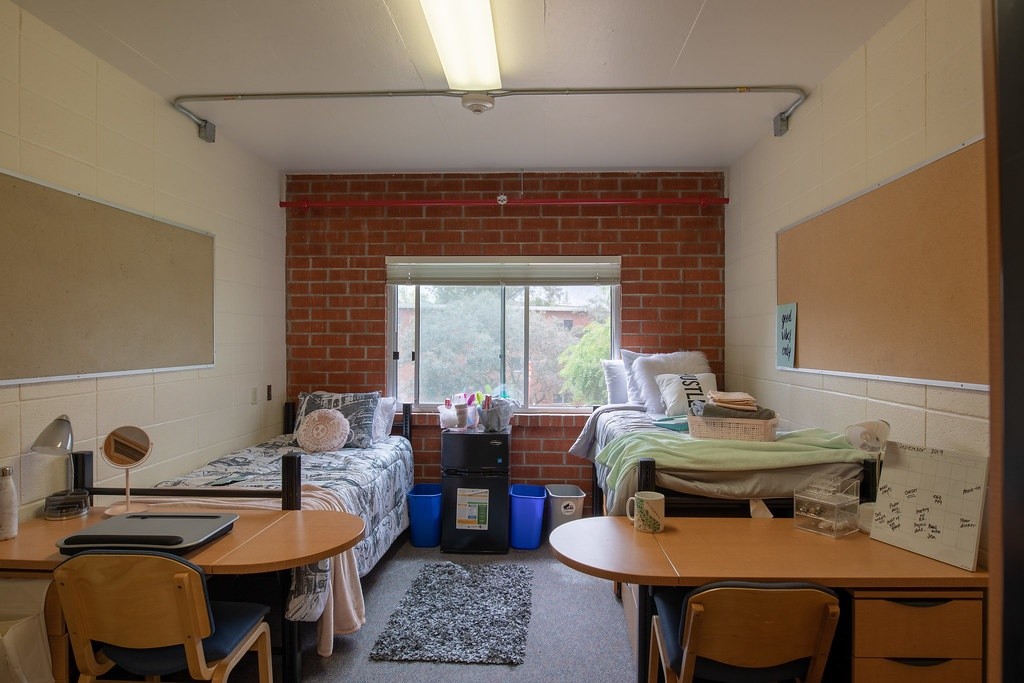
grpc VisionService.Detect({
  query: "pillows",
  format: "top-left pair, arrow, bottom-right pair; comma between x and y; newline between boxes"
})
295,409 -> 349,452
633,351 -> 711,414
599,357 -> 630,404
376,396 -> 397,445
289,391 -> 383,446
296,391 -> 310,424
655,373 -> 717,417
620,349 -> 652,403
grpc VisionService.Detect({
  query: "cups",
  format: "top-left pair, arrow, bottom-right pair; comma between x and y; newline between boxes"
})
627,491 -> 665,533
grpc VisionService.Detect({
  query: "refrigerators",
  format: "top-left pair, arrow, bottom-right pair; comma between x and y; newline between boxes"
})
441,425 -> 512,553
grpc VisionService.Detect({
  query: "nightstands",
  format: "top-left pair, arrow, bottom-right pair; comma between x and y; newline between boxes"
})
440,423 -> 511,555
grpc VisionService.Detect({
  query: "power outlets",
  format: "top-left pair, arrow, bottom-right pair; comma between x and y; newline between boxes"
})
267,384 -> 272,400
251,387 -> 259,405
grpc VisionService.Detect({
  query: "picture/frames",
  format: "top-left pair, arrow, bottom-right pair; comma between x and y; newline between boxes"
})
0,168 -> 217,388
773,130 -> 991,395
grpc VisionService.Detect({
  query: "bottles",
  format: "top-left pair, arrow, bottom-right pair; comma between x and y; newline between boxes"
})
0,467 -> 19,540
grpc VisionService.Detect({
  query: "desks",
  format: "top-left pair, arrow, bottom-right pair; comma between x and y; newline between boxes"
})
548,515 -> 990,683
0,507 -> 368,683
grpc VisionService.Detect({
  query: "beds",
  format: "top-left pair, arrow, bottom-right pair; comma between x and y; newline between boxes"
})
590,405 -> 887,516
154,403 -> 416,683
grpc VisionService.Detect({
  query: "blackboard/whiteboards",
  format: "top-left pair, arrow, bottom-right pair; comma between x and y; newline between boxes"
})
774,132 -> 989,397
0,168 -> 216,385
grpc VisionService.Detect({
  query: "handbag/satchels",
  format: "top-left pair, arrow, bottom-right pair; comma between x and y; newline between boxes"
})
477,398 -> 521,431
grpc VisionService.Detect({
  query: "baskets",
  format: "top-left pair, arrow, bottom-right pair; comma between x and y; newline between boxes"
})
686,413 -> 779,442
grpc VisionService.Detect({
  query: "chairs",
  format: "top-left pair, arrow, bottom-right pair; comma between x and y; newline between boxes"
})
646,581 -> 840,683
52,549 -> 275,683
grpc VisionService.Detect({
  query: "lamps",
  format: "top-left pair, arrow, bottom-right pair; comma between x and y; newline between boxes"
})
846,419 -> 891,535
419,0 -> 507,91
30,414 -> 91,520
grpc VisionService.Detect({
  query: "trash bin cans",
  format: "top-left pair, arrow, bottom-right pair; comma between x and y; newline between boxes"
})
509,483 -> 547,549
545,484 -> 586,537
405,483 -> 443,548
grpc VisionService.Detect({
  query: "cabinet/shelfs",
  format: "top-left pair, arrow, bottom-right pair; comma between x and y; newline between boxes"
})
852,588 -> 984,683
793,474 -> 860,540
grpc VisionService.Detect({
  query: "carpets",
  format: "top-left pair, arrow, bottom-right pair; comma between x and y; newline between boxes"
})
370,561 -> 535,666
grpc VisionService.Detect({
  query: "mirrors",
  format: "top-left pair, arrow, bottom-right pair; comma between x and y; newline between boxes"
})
100,425 -> 153,516
